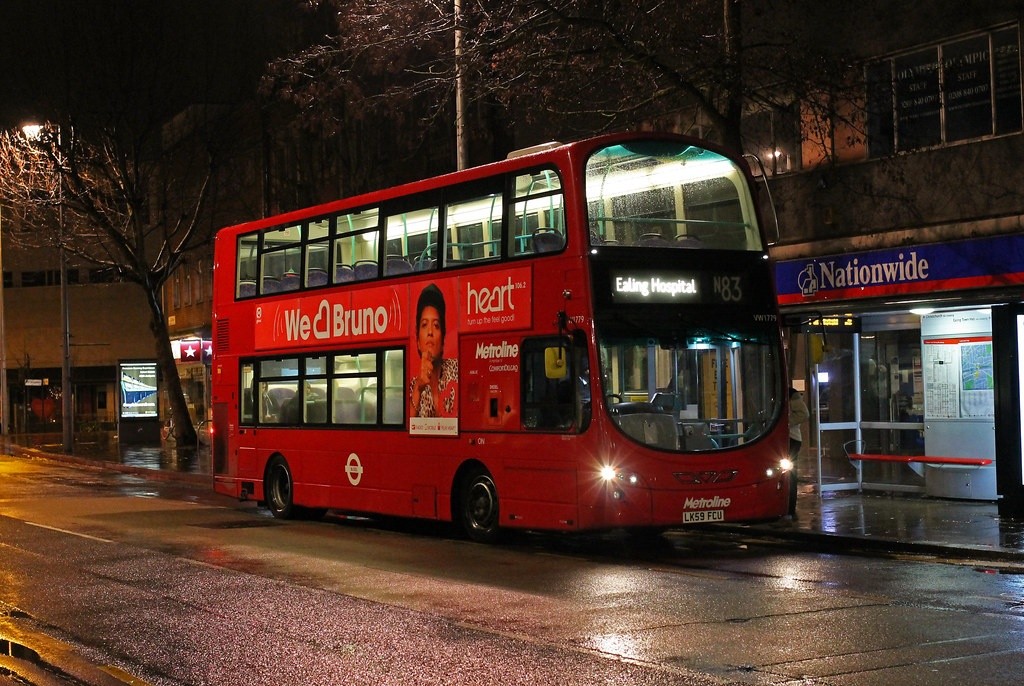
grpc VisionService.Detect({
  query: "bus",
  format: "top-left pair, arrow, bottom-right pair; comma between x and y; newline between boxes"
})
210,129 -> 832,547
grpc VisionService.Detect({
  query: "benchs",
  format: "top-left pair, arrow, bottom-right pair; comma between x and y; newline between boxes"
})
843,440 -> 993,496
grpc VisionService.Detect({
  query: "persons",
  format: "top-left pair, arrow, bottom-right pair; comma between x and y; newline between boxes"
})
770,388 -> 810,528
409,283 -> 459,418
246,377 -> 280,414
283,381 -> 314,423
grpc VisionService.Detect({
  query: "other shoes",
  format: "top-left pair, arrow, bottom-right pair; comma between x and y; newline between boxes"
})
779,511 -> 798,521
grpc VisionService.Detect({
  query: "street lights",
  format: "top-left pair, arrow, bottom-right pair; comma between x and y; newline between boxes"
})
22,122 -> 77,456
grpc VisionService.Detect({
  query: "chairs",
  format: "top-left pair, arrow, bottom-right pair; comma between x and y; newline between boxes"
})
244,388 -> 404,424
238,227 -> 707,298
